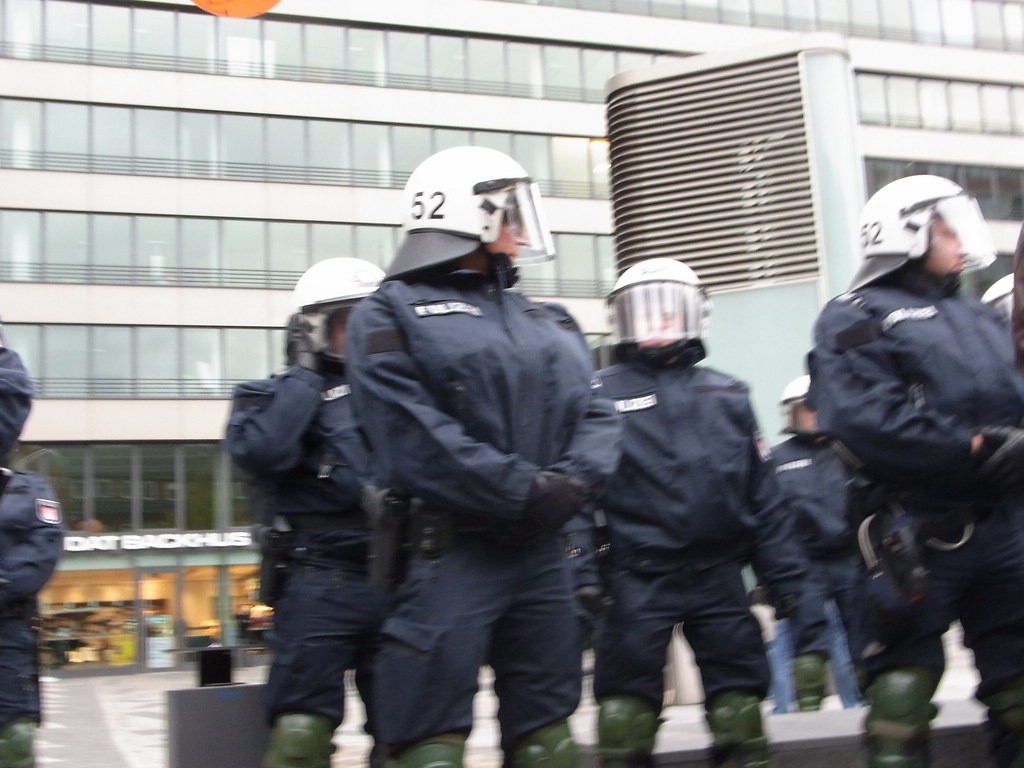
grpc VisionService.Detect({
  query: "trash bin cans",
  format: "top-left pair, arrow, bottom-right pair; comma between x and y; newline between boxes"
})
199,647 -> 232,687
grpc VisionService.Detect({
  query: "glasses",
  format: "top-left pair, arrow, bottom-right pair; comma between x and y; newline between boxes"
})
800,398 -> 818,415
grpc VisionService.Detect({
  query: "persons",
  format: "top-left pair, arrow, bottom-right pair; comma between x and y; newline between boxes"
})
1,348 -> 62,768
222,145 -> 1023,768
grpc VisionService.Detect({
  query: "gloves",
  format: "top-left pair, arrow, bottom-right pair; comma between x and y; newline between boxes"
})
773,592 -> 798,619
523,465 -> 584,532
970,422 -> 1024,485
576,583 -> 617,614
286,313 -> 330,372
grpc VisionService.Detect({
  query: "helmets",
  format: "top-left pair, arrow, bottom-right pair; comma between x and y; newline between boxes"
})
292,258 -> 388,307
780,375 -> 811,403
859,174 -> 964,257
401,142 -> 532,244
606,256 -> 710,318
